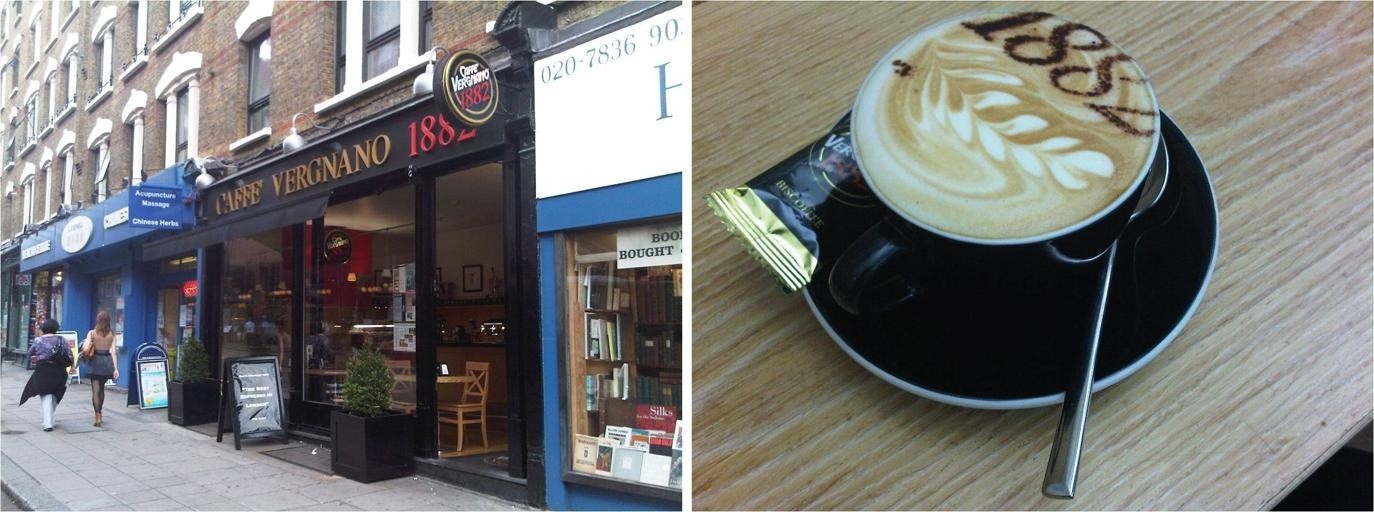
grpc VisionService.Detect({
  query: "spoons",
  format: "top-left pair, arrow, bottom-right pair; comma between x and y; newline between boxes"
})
1039,132 -> 1179,502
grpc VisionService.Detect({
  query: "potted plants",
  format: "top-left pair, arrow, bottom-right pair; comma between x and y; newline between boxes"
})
329,349 -> 416,484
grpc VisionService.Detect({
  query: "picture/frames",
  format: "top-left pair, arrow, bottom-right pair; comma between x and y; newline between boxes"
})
572,434 -> 598,474
605,425 -> 632,447
463,264 -> 483,292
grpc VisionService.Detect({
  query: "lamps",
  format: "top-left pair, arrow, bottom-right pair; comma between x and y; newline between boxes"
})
283,113 -> 333,155
347,273 -> 357,281
413,46 -> 449,94
195,155 -> 239,189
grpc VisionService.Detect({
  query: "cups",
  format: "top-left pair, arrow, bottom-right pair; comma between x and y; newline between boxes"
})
852,12 -> 1161,245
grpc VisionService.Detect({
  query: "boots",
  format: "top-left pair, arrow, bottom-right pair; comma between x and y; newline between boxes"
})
93,412 -> 102,427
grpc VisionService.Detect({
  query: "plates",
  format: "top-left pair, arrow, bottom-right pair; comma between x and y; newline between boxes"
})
776,78 -> 1221,413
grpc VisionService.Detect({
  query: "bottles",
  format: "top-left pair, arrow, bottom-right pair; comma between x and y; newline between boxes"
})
487,265 -> 498,300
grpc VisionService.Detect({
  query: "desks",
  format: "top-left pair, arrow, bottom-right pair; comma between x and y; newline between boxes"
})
394,375 -> 477,454
692,0 -> 1373,511
308,369 -> 348,376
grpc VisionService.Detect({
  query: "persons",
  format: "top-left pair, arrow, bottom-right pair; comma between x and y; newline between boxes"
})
25,318 -> 75,432
223,281 -> 290,371
80,311 -> 119,427
310,327 -> 333,402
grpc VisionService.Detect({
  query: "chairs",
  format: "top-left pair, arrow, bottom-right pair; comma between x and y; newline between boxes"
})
437,361 -> 490,452
382,360 -> 414,401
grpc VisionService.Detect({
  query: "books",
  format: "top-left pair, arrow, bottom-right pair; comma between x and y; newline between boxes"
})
572,261 -> 681,490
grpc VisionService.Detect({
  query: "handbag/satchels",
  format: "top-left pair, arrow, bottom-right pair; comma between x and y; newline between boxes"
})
48,345 -> 75,368
80,338 -> 95,360
